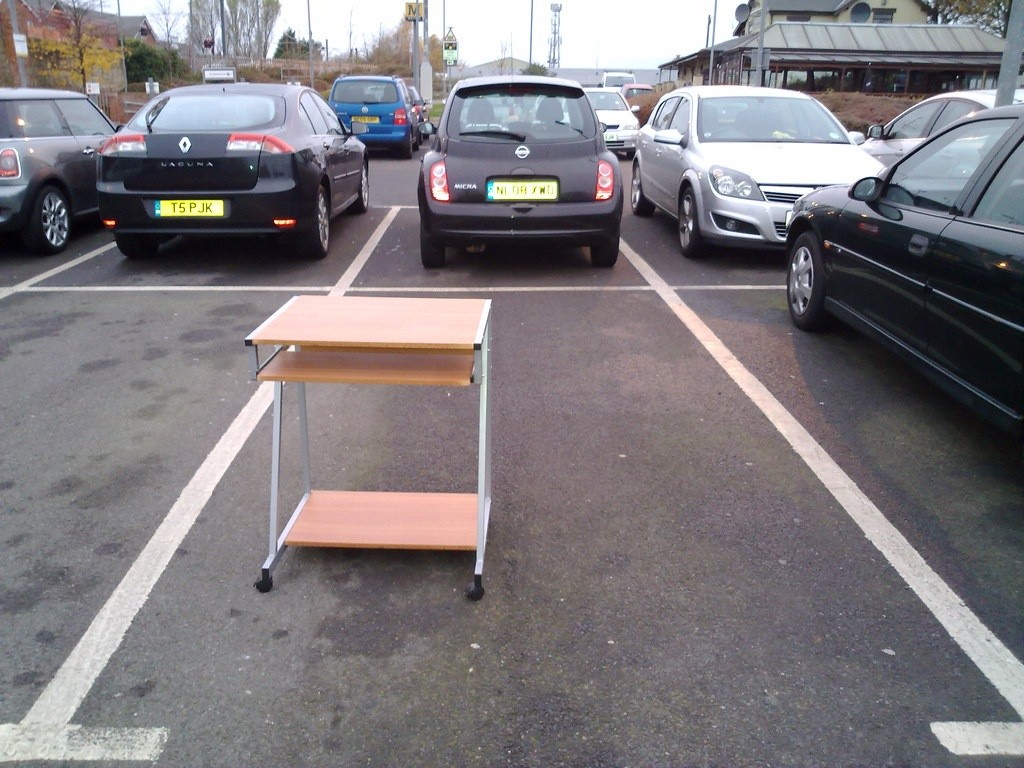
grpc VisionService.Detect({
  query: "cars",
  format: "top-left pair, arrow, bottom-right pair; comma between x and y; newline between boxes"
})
630,84 -> 895,262
620,83 -> 653,101
95,81 -> 371,263
528,86 -> 643,159
0,89 -> 127,257
860,89 -> 1024,169
362,84 -> 434,145
418,74 -> 626,269
784,104 -> 1024,454
324,73 -> 423,161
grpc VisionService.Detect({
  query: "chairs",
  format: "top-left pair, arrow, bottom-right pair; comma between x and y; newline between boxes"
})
25,106 -> 60,137
380,85 -> 397,102
703,103 -> 718,134
531,98 -> 569,135
465,97 -> 502,131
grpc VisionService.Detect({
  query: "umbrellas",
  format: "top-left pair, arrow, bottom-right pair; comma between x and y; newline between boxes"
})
862,62 -> 873,93
955,76 -> 959,92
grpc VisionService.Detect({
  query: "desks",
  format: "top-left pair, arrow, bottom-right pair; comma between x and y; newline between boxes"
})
243,295 -> 492,600
